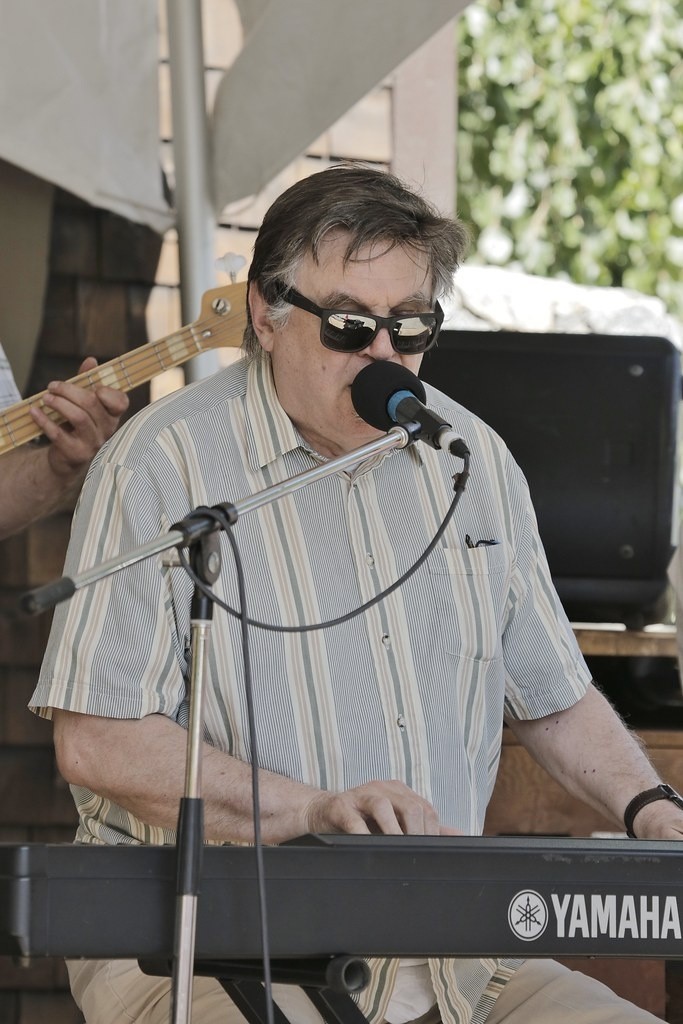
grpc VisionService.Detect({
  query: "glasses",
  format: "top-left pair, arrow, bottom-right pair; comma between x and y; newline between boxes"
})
248,278 -> 444,357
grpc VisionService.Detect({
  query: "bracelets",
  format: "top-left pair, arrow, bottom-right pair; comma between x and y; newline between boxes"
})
624,783 -> 683,839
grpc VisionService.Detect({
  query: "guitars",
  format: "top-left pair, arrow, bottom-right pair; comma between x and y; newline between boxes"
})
0,281 -> 249,459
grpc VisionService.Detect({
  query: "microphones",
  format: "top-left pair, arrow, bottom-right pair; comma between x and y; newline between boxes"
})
351,360 -> 472,460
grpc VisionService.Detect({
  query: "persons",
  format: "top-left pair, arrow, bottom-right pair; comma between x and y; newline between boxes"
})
25,167 -> 683,1023
0,338 -> 129,538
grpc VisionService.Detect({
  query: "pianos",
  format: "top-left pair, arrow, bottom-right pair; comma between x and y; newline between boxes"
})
0,830 -> 683,968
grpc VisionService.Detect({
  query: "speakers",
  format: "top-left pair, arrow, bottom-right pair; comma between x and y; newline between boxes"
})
414,331 -> 679,626
